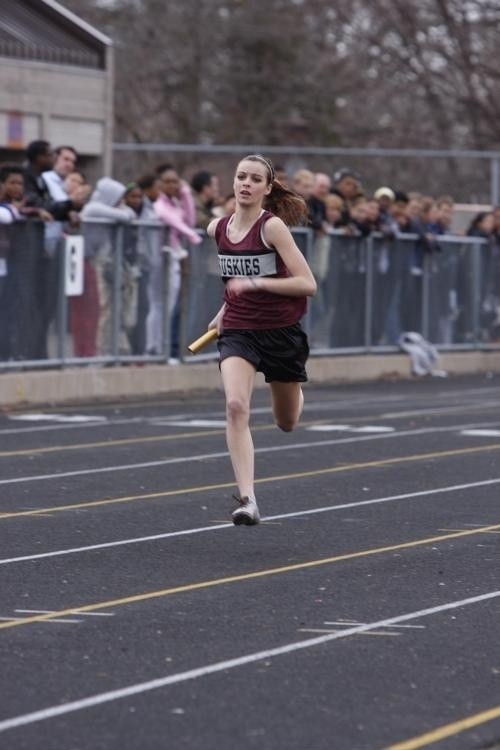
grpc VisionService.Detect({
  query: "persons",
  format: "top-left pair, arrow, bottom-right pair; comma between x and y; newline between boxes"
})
205,152 -> 319,529
1,139 -> 498,371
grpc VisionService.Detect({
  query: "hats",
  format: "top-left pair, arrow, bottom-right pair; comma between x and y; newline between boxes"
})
374,186 -> 395,201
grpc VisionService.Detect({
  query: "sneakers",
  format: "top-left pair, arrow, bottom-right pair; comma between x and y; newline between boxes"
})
230,499 -> 261,524
